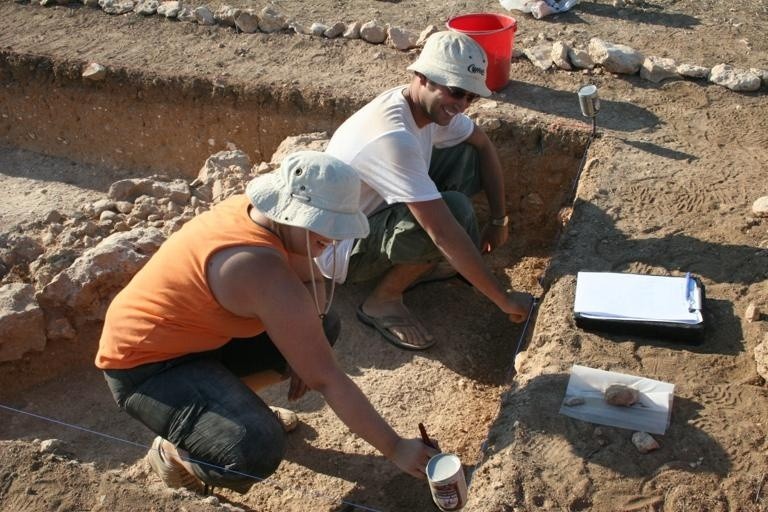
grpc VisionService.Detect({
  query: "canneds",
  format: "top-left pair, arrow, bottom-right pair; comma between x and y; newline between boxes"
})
578,84 -> 600,118
426,453 -> 468,512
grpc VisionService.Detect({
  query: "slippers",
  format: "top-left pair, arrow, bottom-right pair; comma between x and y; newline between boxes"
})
355,299 -> 438,352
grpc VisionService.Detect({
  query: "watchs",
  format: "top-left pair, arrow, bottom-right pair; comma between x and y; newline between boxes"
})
491,216 -> 509,226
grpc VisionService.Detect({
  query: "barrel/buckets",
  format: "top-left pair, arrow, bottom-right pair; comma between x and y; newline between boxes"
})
445,13 -> 518,90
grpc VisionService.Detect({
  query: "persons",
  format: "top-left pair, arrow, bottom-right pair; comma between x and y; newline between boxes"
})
313,31 -> 534,351
95,149 -> 441,494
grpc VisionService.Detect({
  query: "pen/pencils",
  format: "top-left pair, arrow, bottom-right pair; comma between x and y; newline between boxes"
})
418,422 -> 432,446
686,271 -> 692,299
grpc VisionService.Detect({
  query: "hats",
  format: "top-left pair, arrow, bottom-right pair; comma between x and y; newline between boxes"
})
246,150 -> 370,239
406,31 -> 494,98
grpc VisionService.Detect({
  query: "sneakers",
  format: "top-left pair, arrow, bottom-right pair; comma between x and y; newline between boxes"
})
268,405 -> 299,432
147,435 -> 205,495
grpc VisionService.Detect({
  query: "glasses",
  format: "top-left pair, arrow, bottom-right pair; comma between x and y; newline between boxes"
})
447,87 -> 481,104
